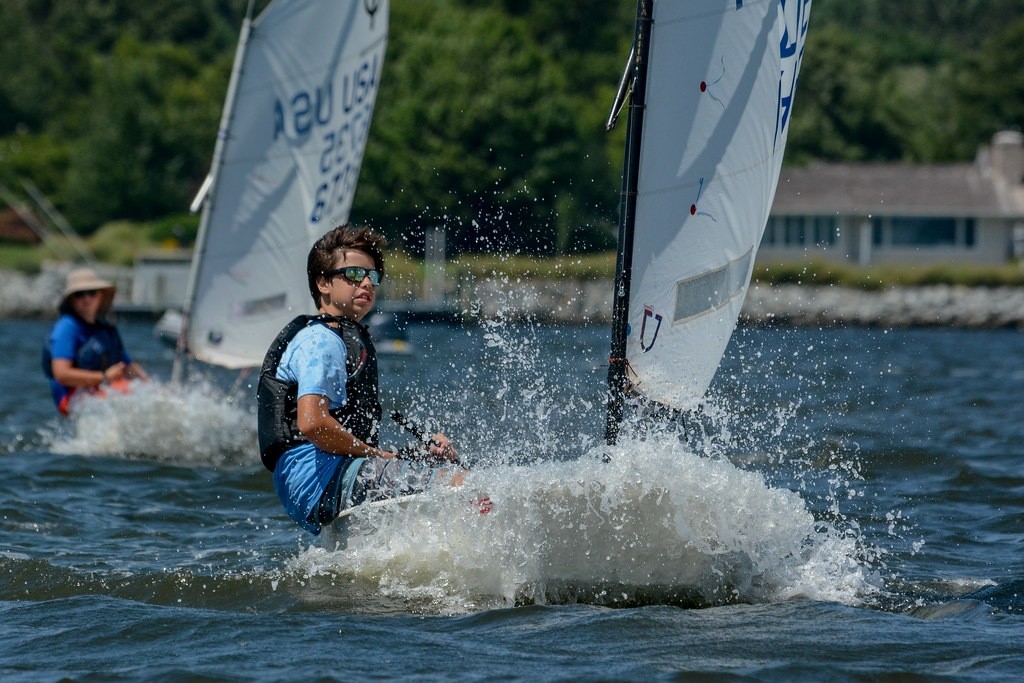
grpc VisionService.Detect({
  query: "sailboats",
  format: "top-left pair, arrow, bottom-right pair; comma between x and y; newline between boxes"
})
300,0 -> 820,610
63,0 -> 391,465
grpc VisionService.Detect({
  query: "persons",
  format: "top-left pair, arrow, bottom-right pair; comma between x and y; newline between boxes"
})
253,223 -> 477,551
40,265 -> 150,422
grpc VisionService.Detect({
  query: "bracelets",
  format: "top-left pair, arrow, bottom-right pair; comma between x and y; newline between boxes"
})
102,370 -> 109,385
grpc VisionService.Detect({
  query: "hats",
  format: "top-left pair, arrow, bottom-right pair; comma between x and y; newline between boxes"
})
52,267 -> 116,315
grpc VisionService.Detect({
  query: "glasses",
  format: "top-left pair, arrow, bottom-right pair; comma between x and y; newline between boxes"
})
69,290 -> 100,299
327,266 -> 384,286
